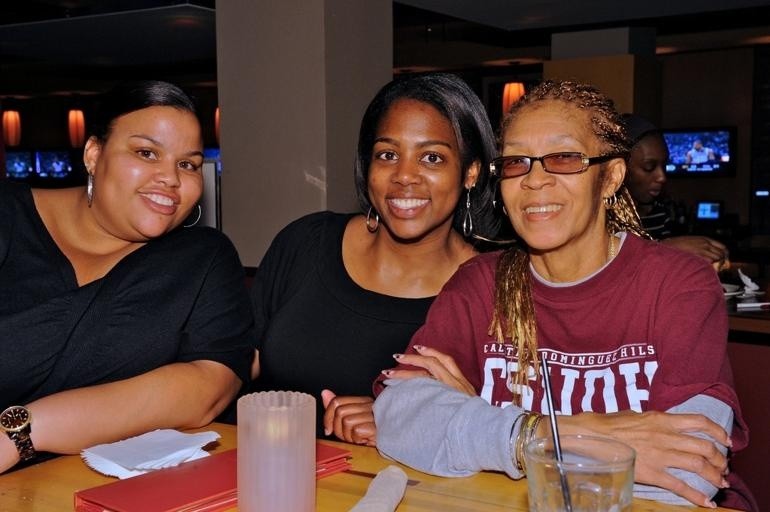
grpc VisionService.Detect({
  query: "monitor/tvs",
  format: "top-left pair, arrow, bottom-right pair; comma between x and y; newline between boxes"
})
35,149 -> 74,179
2,149 -> 35,178
695,199 -> 724,220
662,126 -> 738,182
202,145 -> 221,180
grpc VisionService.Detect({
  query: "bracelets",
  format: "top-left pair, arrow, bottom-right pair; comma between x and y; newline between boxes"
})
508,410 -> 547,478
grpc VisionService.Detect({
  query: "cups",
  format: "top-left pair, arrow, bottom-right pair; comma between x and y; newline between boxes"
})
518,433 -> 634,512
235,388 -> 318,511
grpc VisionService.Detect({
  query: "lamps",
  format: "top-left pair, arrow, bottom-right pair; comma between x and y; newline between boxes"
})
1,96 -> 21,146
67,93 -> 86,148
502,61 -> 526,115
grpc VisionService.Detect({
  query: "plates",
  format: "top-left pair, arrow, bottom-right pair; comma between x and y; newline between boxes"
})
720,283 -> 745,300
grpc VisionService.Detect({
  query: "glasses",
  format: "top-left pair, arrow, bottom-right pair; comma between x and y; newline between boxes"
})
489,152 -> 625,178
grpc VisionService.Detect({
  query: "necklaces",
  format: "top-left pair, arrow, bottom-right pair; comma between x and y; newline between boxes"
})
606,232 -> 618,263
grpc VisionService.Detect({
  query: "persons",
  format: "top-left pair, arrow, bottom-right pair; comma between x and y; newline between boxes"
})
1,81 -> 255,476
213,76 -> 485,449
615,111 -> 730,273
681,139 -> 718,172
371,74 -> 761,512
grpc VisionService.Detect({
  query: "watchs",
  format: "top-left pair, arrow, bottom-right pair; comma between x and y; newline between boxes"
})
0,405 -> 38,464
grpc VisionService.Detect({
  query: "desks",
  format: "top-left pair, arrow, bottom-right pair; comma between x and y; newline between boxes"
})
720,278 -> 770,512
0,421 -> 745,512
